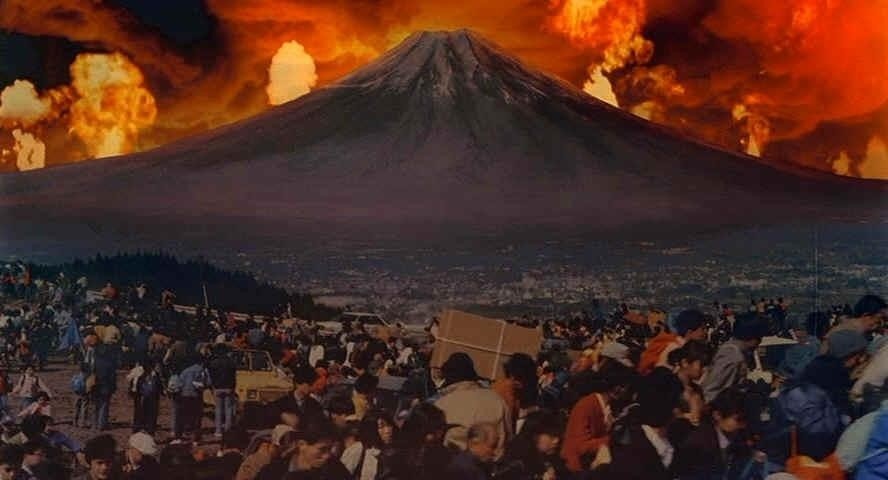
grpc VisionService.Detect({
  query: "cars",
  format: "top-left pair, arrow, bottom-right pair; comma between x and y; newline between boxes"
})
334,312 -> 392,328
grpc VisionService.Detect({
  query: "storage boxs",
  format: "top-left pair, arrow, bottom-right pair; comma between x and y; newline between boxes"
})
426,308 -> 543,385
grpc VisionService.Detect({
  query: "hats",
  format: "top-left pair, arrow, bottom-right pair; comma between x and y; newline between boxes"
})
828,330 -> 869,360
441,353 -> 478,379
599,342 -> 635,367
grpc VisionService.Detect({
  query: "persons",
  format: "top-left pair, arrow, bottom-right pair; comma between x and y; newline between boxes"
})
1,260 -> 888,479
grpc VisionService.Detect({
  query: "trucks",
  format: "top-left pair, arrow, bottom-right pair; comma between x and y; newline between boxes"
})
203,344 -> 296,412
